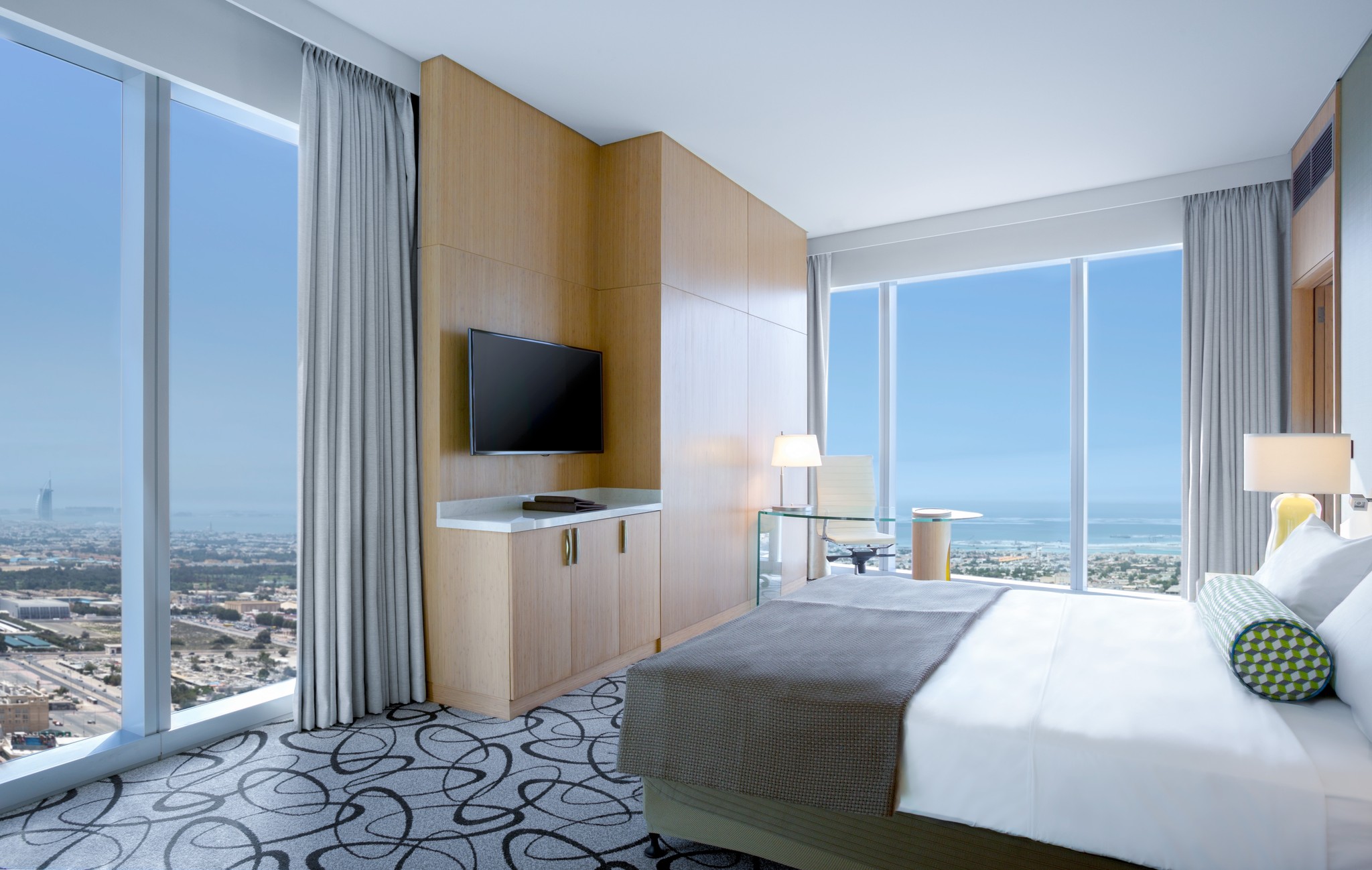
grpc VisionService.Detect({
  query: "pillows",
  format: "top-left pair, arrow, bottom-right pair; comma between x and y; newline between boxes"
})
1252,513 -> 1372,630
1195,575 -> 1335,704
1315,570 -> 1371,746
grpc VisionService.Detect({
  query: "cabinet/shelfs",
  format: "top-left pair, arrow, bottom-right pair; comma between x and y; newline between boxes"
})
510,519 -> 620,702
619,510 -> 662,654
660,134 -> 807,637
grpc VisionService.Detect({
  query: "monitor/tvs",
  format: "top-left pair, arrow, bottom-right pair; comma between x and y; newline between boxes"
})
468,327 -> 603,456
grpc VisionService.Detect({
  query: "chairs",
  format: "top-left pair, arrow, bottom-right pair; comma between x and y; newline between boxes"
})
817,455 -> 898,572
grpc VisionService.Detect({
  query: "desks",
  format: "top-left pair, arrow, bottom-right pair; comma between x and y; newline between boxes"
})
757,506 -> 983,608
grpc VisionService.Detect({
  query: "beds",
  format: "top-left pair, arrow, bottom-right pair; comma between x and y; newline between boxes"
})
617,575 -> 1372,870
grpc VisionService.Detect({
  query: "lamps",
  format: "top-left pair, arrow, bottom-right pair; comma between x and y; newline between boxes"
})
770,430 -> 822,512
1347,493 -> 1372,511
1243,432 -> 1352,566
